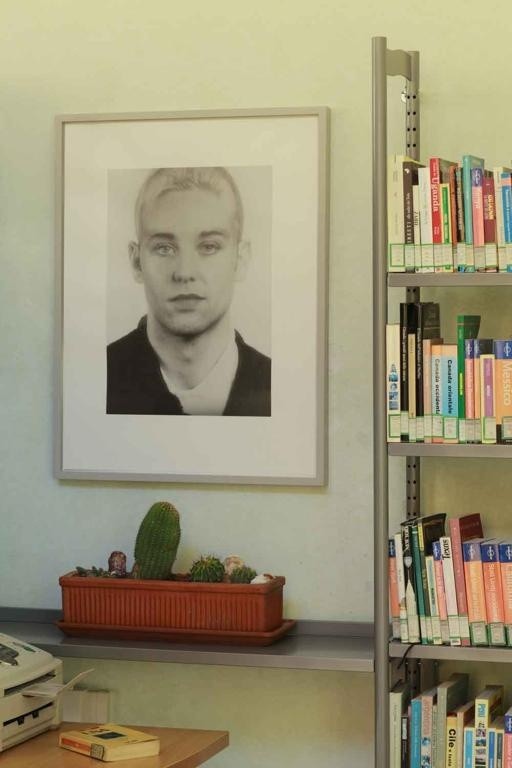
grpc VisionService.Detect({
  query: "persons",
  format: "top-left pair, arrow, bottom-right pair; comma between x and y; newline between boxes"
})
107,167 -> 272,416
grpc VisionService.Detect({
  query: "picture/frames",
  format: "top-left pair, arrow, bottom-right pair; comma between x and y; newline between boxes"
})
48,109 -> 333,490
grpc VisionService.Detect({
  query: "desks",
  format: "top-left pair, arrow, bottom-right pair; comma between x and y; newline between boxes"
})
0,720 -> 229,768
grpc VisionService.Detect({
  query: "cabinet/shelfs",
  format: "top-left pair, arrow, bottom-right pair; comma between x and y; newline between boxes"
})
369,33 -> 512,768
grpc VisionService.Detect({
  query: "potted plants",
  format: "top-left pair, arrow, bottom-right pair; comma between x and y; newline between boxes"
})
55,500 -> 302,647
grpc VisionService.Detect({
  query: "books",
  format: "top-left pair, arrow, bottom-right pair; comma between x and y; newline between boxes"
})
388,673 -> 512,768
386,156 -> 512,273
388,512 -> 512,647
58,720 -> 164,762
385,298 -> 512,445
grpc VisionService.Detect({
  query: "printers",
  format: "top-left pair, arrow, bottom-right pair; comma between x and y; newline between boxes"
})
1,634 -> 63,755
